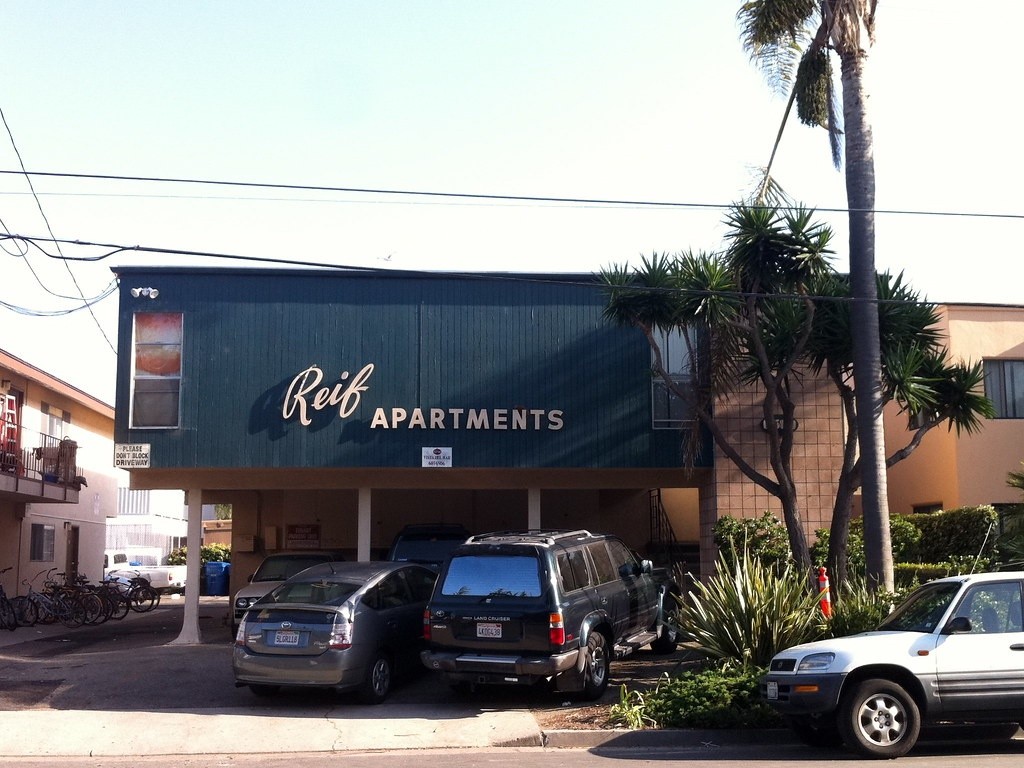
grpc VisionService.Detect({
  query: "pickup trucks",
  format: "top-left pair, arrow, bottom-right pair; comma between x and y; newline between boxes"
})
104,549 -> 188,592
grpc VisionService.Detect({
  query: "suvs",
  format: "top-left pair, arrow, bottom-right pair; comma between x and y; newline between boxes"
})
420,530 -> 684,699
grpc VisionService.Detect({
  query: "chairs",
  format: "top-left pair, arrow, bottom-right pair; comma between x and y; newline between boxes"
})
1008,600 -> 1023,633
979,606 -> 1001,634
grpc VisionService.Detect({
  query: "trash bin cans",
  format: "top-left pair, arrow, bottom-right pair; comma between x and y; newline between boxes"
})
205,561 -> 230,596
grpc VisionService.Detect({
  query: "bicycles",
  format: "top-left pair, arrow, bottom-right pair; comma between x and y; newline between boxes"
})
0,566 -> 160,632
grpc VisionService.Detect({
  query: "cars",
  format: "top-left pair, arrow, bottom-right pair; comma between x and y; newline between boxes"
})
389,521 -> 475,573
227,549 -> 343,643
230,561 -> 438,704
762,570 -> 1024,755
